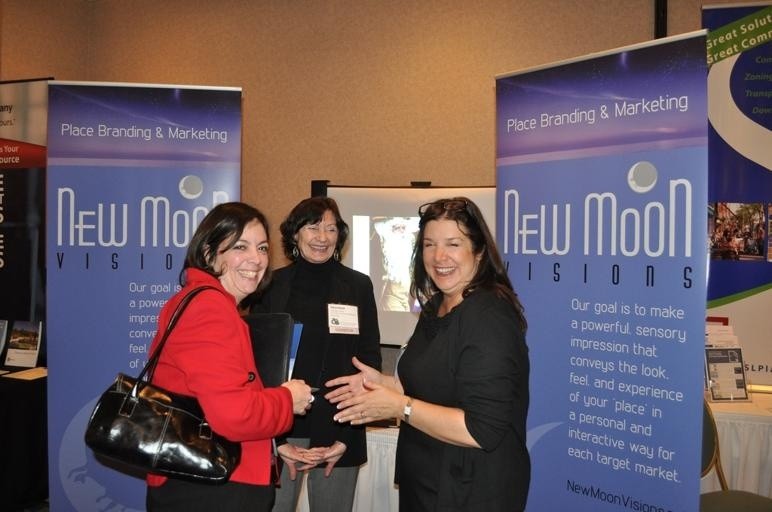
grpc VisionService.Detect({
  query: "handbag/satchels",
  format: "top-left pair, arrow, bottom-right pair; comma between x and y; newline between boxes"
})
85,372 -> 241,487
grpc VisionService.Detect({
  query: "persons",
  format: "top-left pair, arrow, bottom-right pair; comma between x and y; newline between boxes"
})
240,193 -> 383,511
710,215 -> 764,262
320,194 -> 534,511
136,201 -> 316,511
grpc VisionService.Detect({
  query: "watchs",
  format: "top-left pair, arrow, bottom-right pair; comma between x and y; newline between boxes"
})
402,398 -> 414,424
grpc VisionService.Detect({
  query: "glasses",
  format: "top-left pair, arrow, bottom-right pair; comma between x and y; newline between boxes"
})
418,199 -> 467,218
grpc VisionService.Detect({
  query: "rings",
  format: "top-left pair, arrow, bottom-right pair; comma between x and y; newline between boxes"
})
360,412 -> 365,418
308,394 -> 316,404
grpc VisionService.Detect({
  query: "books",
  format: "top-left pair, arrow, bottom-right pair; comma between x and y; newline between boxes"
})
0,319 -> 9,358
3,319 -> 43,368
704,324 -> 748,402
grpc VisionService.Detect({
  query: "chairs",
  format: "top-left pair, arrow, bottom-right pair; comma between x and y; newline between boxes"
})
699,397 -> 772,512
